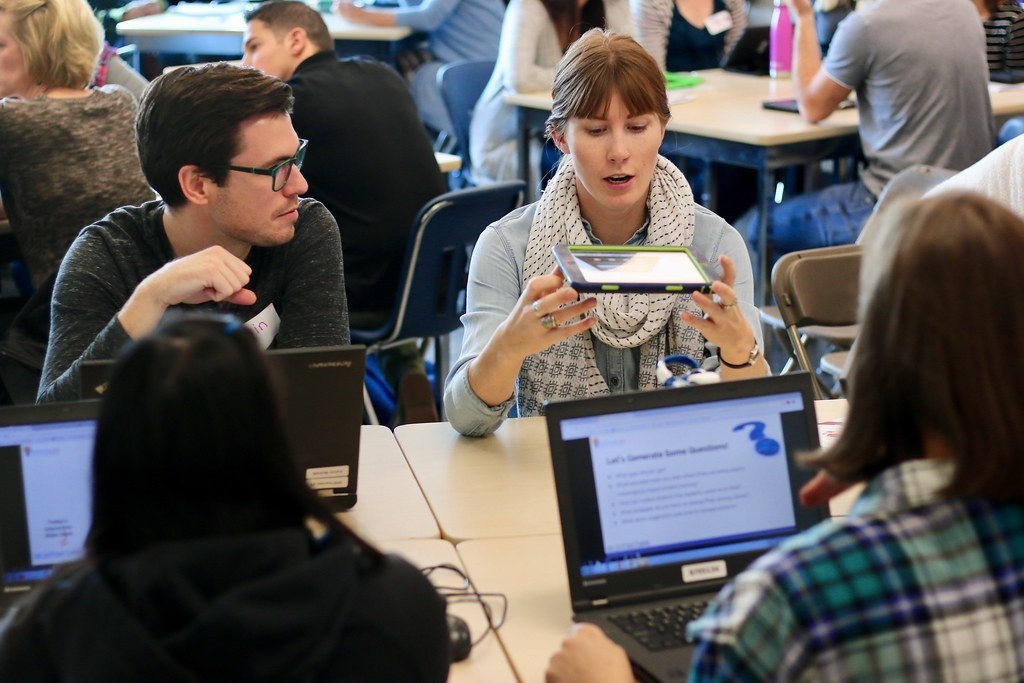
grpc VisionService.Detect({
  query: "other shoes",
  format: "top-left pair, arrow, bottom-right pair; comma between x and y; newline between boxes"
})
399,368 -> 438,424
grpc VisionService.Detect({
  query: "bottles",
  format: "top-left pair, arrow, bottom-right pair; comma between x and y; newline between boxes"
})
769,1 -> 793,79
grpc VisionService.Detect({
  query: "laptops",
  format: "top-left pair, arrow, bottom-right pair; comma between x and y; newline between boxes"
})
542,370 -> 831,683
79,344 -> 364,513
0,397 -> 99,623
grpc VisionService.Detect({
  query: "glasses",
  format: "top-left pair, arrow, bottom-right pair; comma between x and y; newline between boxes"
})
224,138 -> 309,191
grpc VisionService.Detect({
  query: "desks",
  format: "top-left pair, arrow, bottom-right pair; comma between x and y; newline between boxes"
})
502,67 -> 1023,311
115,4 -> 420,74
330,400 -> 867,683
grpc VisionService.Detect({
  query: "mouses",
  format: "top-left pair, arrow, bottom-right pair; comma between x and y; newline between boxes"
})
446,613 -> 473,662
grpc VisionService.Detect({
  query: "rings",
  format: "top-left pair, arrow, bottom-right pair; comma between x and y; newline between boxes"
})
532,301 -> 547,317
541,313 -> 559,330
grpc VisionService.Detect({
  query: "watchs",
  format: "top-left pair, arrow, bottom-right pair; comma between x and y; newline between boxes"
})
716,336 -> 761,370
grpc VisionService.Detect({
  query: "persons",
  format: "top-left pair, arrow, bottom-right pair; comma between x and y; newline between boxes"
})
0,0 -> 1024,433
544,189 -> 1024,683
0,308 -> 450,683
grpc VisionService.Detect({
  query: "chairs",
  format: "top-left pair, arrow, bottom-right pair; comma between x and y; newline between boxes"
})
330,176 -> 526,427
770,244 -> 865,401
433,60 -> 499,164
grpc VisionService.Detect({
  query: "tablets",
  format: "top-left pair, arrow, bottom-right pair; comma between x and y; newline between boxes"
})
763,99 -> 857,113
551,245 -> 713,295
